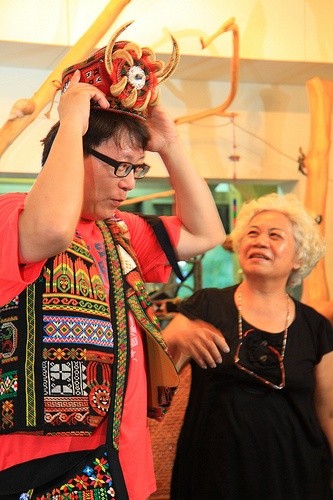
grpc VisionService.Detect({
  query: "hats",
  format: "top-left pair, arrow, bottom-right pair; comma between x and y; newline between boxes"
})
61,19 -> 181,122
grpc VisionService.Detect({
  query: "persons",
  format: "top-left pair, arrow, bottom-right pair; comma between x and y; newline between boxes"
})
0,69 -> 333,500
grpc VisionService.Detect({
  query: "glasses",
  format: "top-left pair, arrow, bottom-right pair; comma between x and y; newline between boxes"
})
234,328 -> 285,389
86,147 -> 150,180
